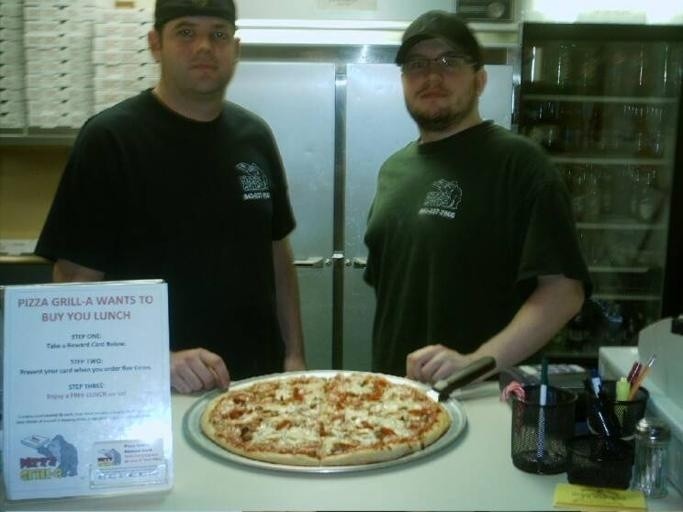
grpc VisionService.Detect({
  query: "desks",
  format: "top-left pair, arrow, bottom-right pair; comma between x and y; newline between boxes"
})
0,381 -> 569,512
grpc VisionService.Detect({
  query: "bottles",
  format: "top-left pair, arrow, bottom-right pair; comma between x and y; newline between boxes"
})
631,418 -> 670,498
521,34 -> 678,268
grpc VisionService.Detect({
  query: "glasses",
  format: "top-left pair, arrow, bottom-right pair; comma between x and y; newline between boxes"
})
401,55 -> 476,70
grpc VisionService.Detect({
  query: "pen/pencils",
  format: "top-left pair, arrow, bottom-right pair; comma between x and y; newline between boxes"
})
590,370 -> 611,436
539,357 -> 548,461
629,356 -> 656,400
628,362 -> 642,385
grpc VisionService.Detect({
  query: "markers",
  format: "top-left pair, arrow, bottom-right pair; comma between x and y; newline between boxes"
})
615,377 -> 630,427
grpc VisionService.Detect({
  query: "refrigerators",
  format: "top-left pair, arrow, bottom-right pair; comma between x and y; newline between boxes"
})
229,18 -> 520,370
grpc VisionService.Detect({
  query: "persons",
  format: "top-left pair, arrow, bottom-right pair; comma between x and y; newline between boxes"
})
36,0 -> 309,395
362,9 -> 593,393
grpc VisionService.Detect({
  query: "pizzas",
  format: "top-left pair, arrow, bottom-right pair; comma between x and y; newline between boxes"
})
199,372 -> 451,466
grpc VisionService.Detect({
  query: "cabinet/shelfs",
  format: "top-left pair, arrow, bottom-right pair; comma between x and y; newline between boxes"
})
515,21 -> 682,357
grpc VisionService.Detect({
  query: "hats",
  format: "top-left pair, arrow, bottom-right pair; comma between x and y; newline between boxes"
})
395,10 -> 478,65
155,0 -> 234,29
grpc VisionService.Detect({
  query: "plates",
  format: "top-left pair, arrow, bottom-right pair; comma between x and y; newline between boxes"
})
182,368 -> 465,474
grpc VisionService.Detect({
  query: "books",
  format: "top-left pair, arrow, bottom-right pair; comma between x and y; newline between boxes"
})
0,278 -> 172,503
